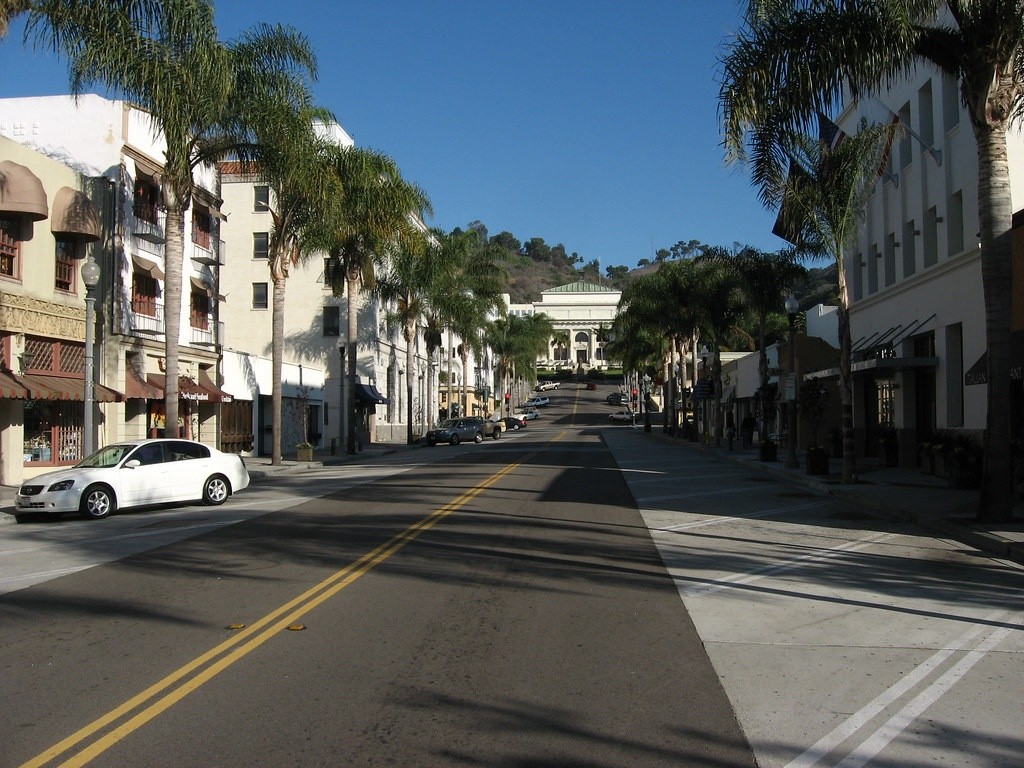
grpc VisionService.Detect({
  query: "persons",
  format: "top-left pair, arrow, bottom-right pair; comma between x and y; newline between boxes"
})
119,446 -> 144,464
832,428 -> 841,458
843,430 -> 857,484
878,432 -> 886,464
727,412 -> 756,448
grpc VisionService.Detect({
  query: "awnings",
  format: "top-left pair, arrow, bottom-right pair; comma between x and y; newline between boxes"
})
190,276 -> 227,314
712,385 -> 733,403
135,160 -> 160,193
22,374 -> 122,403
51,186 -> 100,243
0,370 -> 53,400
147,373 -> 212,400
192,193 -> 227,232
131,253 -> 164,297
125,358 -> 164,399
965,330 -> 1024,385
0,160 -> 48,221
355,383 -> 389,404
199,366 -> 233,402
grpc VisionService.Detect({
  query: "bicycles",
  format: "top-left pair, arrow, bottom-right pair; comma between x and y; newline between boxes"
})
741,426 -> 754,449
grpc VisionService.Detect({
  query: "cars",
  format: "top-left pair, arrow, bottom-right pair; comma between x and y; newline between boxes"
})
15,440 -> 251,521
607,391 -> 629,407
511,395 -> 550,422
587,382 -> 596,390
480,419 -> 502,440
494,417 -> 527,430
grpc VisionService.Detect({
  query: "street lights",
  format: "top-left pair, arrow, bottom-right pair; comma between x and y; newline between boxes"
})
784,293 -> 800,466
81,255 -> 101,459
700,345 -> 711,446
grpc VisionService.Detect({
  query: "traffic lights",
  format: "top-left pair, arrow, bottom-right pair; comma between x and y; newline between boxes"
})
505,393 -> 510,404
633,389 -> 638,400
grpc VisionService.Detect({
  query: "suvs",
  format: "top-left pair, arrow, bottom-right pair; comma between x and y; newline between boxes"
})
425,417 -> 484,447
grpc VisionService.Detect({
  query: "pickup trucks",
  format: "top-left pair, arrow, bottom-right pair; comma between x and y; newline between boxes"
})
609,411 -> 640,425
535,380 -> 560,392
